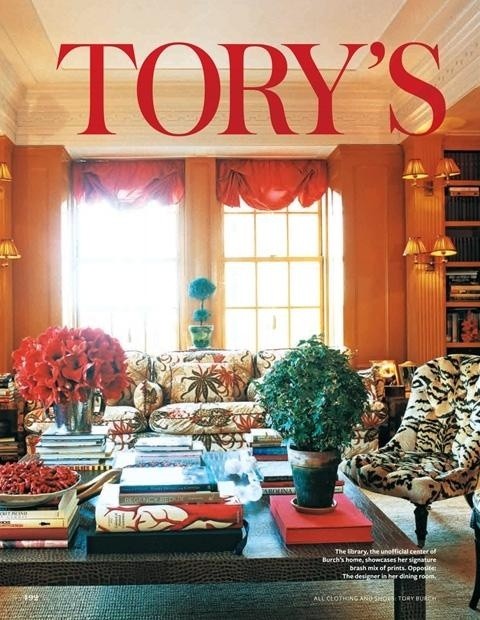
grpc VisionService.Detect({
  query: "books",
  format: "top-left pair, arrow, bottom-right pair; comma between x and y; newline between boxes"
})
0,372 -> 19,404
0,422 -> 375,555
444,150 -> 480,343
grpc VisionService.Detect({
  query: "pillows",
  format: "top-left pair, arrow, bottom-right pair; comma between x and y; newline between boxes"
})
167,362 -> 238,403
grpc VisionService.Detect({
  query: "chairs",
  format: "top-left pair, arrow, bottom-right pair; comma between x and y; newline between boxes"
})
345,353 -> 479,612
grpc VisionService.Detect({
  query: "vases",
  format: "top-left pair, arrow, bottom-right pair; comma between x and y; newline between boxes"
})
43,382 -> 107,436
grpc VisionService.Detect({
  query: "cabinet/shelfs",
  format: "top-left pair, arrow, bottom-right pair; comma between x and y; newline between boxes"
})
403,151 -> 479,365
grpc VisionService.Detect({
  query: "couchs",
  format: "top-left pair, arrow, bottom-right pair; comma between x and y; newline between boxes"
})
28,346 -> 391,458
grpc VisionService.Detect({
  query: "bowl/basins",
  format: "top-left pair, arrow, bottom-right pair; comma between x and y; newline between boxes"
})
1,465 -> 81,510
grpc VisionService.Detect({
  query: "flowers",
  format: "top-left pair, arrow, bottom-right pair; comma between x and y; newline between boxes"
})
12,325 -> 129,412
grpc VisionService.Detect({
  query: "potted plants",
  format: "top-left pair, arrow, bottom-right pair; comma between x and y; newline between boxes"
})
251,331 -> 371,515
187,276 -> 216,349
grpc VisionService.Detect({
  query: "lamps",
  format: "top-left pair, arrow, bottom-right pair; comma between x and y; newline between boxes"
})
0,235 -> 20,268
402,158 -> 462,195
0,161 -> 12,202
402,233 -> 458,272
